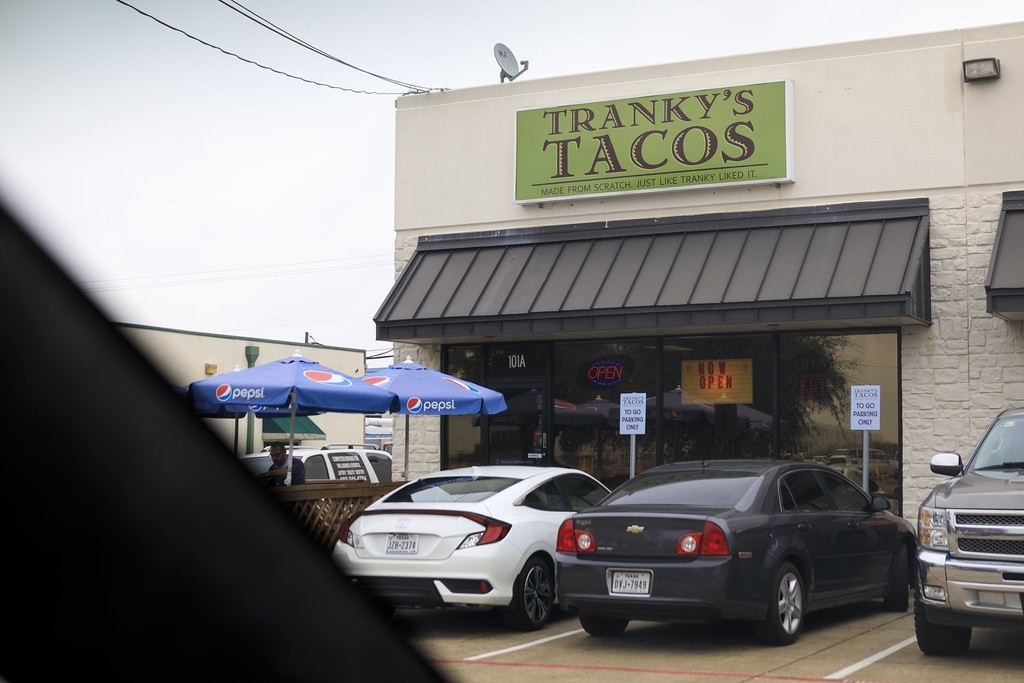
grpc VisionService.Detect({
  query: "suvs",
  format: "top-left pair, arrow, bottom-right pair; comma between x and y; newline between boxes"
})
241,443 -> 393,487
913,404 -> 1024,662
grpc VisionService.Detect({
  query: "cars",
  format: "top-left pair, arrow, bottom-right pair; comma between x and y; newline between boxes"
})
556,459 -> 919,646
787,444 -> 898,509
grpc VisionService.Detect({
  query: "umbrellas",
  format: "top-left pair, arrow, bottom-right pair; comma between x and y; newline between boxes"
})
189,349 -> 402,486
356,354 -> 510,483
182,362 -> 328,461
472,385 -> 774,433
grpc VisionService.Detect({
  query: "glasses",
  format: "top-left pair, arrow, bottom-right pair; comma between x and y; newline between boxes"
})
268,450 -> 284,458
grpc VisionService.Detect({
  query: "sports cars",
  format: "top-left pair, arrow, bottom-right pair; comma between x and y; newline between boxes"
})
327,465 -> 616,629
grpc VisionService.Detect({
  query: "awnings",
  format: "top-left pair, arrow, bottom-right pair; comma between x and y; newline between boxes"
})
262,417 -> 326,440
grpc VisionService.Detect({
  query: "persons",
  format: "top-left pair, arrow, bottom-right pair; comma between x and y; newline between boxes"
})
268,441 -> 306,486
